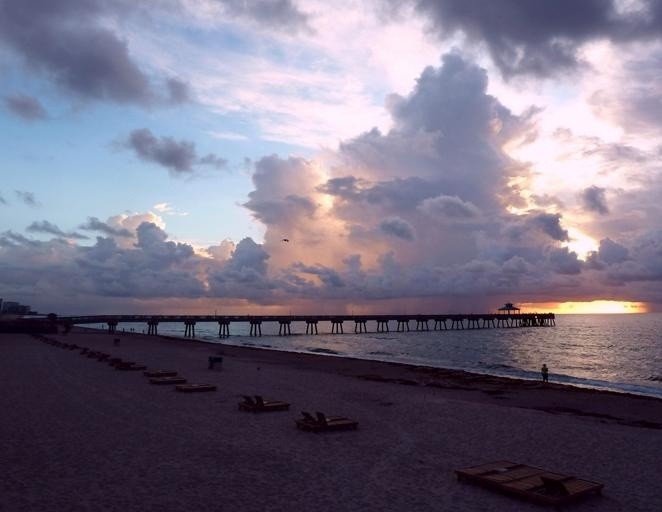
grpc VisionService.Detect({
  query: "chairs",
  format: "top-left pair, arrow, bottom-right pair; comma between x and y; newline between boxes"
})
36,331 -> 217,393
237,394 -> 291,415
292,410 -> 359,433
454,459 -> 604,510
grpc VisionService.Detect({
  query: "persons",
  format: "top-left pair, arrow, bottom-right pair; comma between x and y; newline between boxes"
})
541,364 -> 548,383
